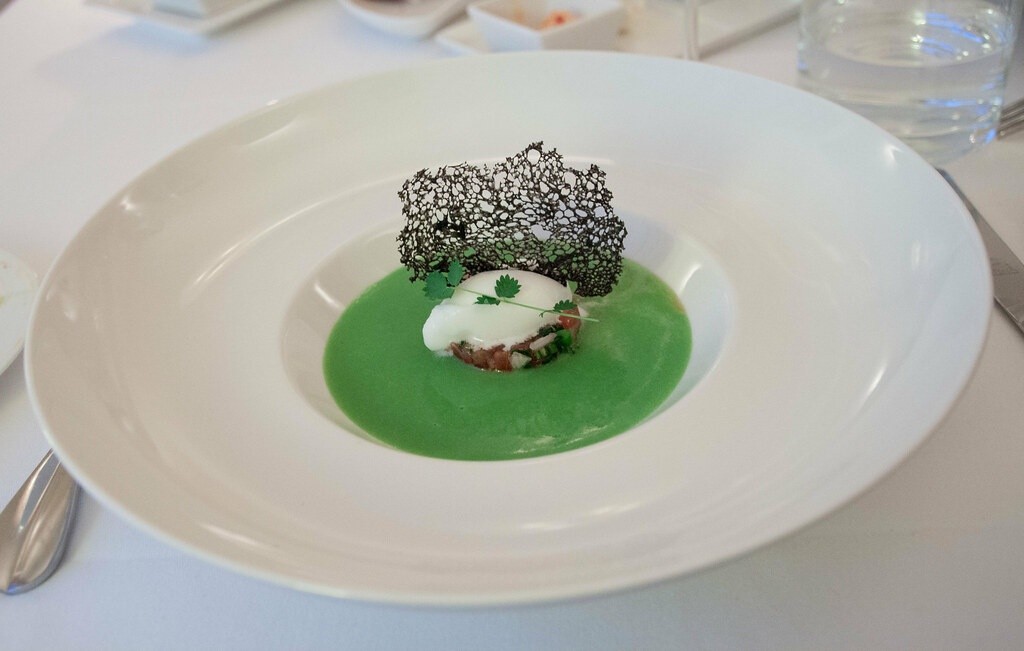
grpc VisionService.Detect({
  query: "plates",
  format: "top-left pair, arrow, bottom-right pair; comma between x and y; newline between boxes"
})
25,48 -> 994,604
83,0 -> 281,36
339,0 -> 465,38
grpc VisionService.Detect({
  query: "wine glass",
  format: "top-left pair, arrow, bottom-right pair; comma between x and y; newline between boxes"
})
675,0 -> 711,64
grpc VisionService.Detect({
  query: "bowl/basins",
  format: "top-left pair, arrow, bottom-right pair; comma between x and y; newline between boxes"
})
467,0 -> 624,49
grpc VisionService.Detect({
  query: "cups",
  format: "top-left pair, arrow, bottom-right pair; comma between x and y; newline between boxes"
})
796,0 -> 1021,169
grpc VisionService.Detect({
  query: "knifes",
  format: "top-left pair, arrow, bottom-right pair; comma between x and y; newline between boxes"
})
933,167 -> 1024,332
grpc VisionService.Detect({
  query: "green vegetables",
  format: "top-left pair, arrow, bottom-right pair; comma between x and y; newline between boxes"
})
423,261 -> 600,366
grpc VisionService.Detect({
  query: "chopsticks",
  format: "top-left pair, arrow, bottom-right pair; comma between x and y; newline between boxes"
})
998,98 -> 1024,137
